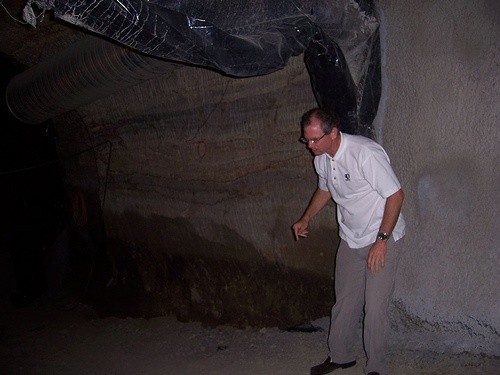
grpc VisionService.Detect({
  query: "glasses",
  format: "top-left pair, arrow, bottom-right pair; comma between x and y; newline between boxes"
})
300,131 -> 327,144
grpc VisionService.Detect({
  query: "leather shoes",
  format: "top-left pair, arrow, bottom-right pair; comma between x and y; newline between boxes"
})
310,356 -> 356,375
368,371 -> 379,375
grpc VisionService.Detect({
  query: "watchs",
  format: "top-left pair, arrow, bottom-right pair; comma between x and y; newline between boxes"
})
378,232 -> 390,241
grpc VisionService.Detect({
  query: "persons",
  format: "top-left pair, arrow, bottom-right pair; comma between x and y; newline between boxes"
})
292,108 -> 407,375
